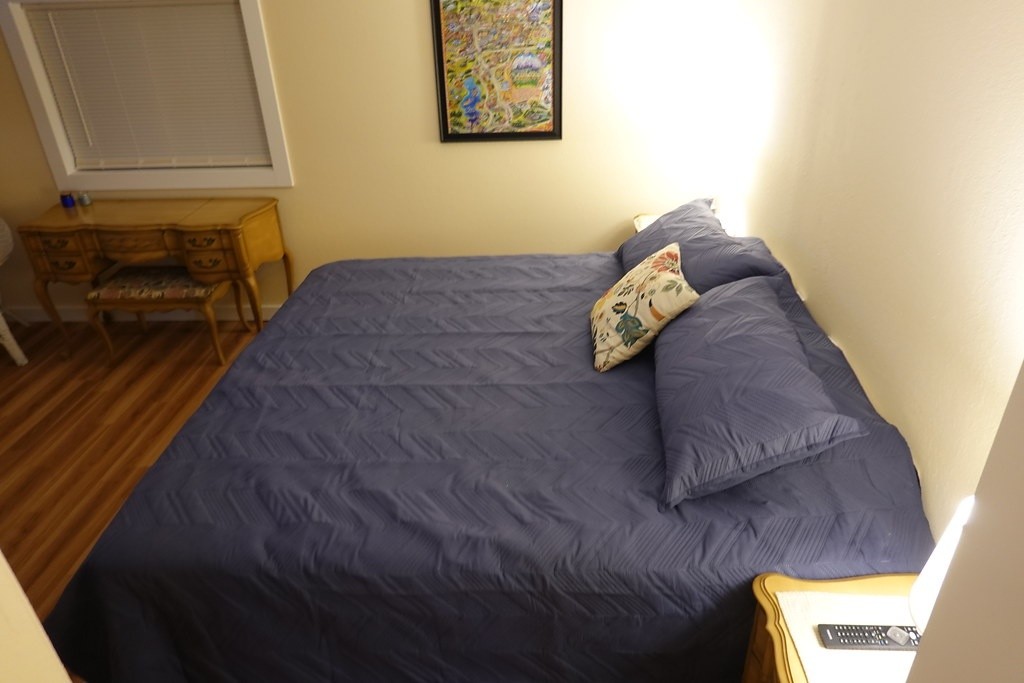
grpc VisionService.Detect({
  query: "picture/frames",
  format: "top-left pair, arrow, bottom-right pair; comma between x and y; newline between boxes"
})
430,0 -> 562,142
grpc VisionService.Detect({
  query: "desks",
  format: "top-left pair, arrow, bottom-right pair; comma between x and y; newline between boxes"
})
630,210 -> 742,237
15,194 -> 292,340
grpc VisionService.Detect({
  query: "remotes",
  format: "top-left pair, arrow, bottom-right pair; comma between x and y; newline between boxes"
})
817,623 -> 922,651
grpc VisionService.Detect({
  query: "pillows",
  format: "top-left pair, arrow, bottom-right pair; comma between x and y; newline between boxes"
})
613,197 -> 786,295
589,241 -> 700,373
651,271 -> 870,511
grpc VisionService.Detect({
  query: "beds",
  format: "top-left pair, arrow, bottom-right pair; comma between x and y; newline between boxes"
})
42,249 -> 934,682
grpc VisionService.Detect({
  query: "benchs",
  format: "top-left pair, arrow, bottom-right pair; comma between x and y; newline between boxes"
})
86,270 -> 254,367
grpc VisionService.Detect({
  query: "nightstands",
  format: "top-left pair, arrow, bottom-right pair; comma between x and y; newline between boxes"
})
740,571 -> 921,683
